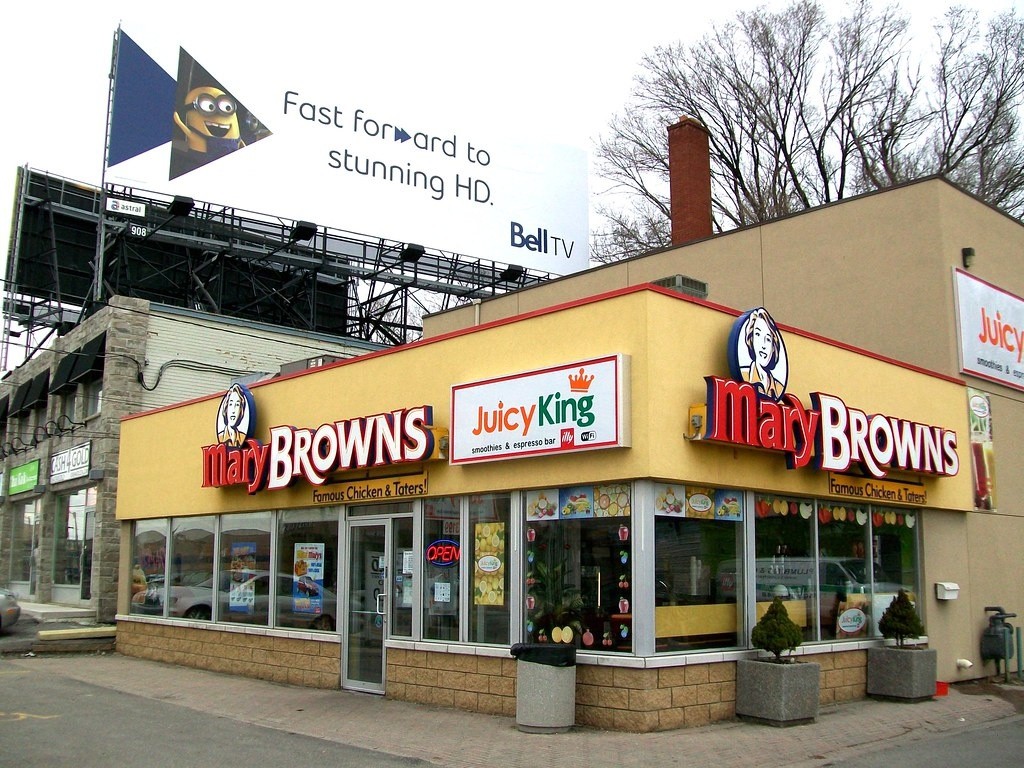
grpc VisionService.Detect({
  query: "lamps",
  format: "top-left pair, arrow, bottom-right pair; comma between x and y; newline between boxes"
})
291,221 -> 318,241
962,247 -> 976,267
500,264 -> 523,282
399,242 -> 425,263
165,194 -> 195,217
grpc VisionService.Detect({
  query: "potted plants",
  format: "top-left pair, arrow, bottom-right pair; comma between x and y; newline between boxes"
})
862,587 -> 938,705
737,595 -> 821,727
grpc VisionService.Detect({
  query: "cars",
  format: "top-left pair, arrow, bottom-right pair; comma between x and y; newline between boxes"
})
130,568 -> 365,635
0,585 -> 21,632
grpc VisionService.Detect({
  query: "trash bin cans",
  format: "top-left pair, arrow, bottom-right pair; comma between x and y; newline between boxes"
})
514,643 -> 576,734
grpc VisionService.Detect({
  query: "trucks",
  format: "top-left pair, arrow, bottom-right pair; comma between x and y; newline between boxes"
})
715,556 -> 916,630
